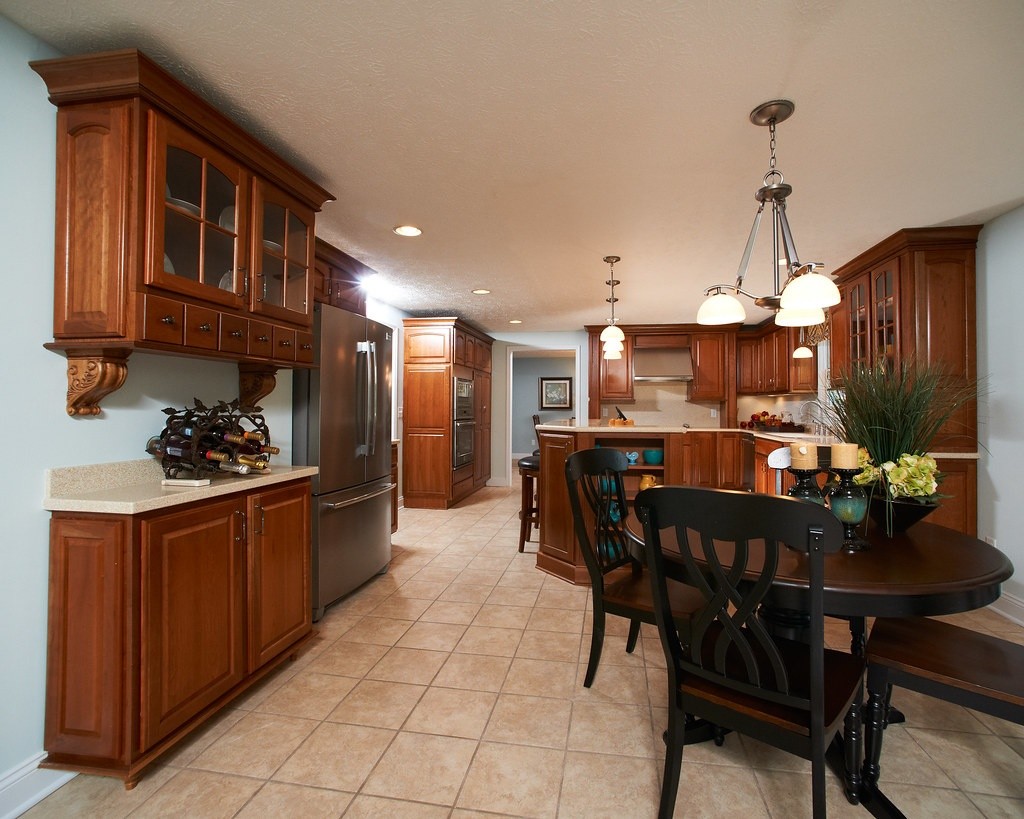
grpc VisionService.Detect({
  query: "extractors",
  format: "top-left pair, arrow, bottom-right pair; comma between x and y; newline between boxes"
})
632,348 -> 694,383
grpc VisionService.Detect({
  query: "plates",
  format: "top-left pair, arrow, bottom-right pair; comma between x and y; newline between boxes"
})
164,252 -> 175,275
262,240 -> 283,254
166,196 -> 200,216
218,205 -> 235,233
219,270 -> 232,292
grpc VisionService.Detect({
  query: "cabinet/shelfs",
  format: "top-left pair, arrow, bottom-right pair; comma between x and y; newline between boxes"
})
531,431 -> 976,585
314,237 -> 379,318
27,47 -> 337,416
582,222 -> 983,401
36,477 -> 320,778
401,317 -> 496,510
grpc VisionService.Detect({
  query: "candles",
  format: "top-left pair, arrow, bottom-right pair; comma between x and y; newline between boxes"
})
830,441 -> 859,469
790,440 -> 818,469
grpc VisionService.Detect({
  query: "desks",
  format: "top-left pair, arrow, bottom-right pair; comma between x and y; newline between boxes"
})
617,502 -> 1014,819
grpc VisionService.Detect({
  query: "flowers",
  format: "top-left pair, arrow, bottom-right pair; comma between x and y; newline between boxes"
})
804,332 -> 995,539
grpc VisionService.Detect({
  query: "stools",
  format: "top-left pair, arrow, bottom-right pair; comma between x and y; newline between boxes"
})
517,448 -> 540,554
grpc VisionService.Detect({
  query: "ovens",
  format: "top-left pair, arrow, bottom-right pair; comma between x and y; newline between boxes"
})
453,376 -> 477,467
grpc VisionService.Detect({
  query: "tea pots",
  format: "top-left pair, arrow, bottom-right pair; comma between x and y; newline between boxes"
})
601,500 -> 620,526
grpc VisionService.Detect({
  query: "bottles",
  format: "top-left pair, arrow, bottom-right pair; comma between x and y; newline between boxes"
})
146,420 -> 280,475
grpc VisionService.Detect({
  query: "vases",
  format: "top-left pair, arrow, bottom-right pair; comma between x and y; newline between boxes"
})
865,493 -> 941,535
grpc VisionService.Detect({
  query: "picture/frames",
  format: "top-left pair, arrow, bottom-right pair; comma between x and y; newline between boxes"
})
538,377 -> 572,410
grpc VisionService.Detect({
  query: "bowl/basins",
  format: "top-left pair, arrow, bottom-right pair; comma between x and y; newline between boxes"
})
602,542 -> 620,558
643,450 -> 663,465
601,479 -> 616,495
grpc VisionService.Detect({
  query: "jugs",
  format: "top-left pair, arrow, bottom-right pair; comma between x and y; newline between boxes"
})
639,474 -> 656,491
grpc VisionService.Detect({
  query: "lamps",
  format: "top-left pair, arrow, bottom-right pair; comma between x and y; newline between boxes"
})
600,256 -> 625,359
696,100 -> 843,328
792,332 -> 831,359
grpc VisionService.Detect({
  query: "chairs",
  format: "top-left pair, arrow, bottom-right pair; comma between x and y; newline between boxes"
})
634,485 -> 867,819
865,613 -> 1024,785
564,447 -> 728,688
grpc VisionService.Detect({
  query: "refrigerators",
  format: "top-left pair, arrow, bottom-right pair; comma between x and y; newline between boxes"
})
292,302 -> 397,622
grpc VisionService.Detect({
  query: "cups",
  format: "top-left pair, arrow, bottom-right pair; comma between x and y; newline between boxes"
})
608,418 -> 634,426
